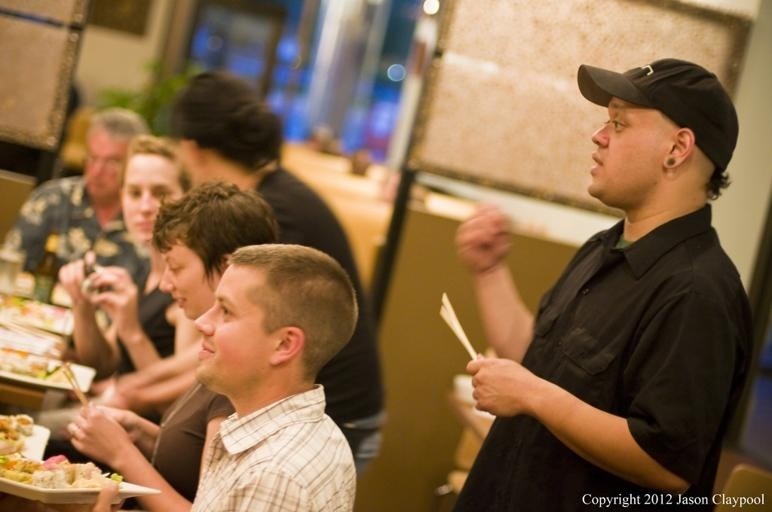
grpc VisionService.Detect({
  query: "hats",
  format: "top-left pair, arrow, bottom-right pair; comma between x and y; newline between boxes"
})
578,59 -> 739,170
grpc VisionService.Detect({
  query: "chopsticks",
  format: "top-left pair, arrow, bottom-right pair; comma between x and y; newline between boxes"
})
63,364 -> 88,407
440,292 -> 477,360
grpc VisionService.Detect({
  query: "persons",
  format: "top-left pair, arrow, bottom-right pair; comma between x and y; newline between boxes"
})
92,244 -> 358,512
452,57 -> 753,512
65,179 -> 279,512
56,136 -> 194,415
2,107 -> 149,290
86,74 -> 386,475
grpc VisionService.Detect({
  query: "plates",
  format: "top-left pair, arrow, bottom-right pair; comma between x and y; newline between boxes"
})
1,461 -> 162,504
19,300 -> 74,337
9,417 -> 51,461
0,353 -> 97,394
